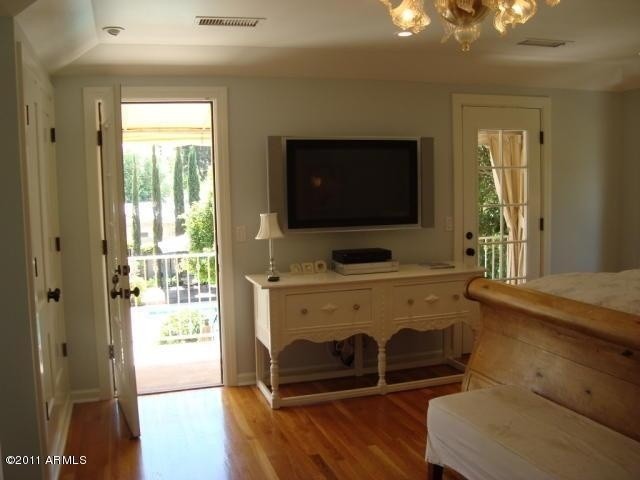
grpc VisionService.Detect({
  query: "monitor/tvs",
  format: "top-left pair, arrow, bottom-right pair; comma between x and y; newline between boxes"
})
280,135 -> 422,233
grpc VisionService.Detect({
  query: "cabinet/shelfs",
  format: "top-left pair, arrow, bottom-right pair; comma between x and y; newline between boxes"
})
240,261 -> 490,410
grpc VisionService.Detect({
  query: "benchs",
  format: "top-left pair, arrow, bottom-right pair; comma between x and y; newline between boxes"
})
423,380 -> 640,480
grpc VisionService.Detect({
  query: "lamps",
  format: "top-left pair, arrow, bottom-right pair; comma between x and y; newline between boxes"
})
379,1 -> 561,52
254,211 -> 287,282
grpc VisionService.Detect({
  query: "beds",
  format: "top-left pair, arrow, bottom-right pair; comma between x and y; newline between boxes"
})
460,267 -> 640,442
